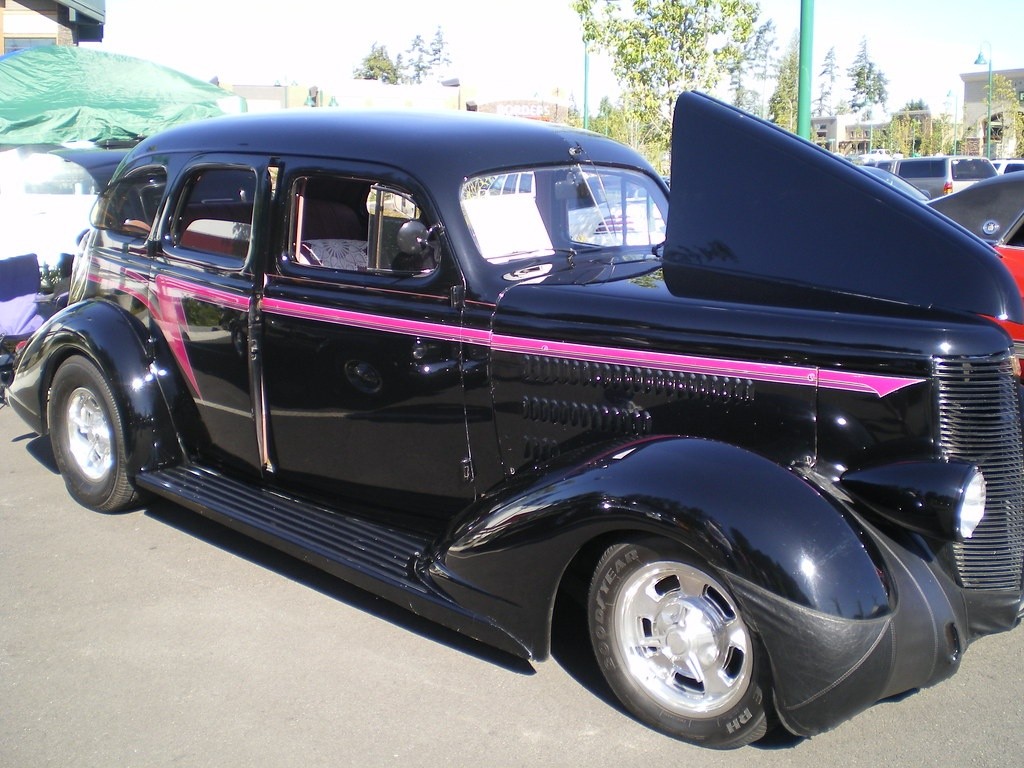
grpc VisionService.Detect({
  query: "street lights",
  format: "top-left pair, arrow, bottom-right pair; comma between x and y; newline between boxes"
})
973,39 -> 992,160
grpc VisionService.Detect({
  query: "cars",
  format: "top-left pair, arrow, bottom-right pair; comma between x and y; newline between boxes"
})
912,175 -> 1024,386
991,159 -> 1024,175
458,165 -> 670,260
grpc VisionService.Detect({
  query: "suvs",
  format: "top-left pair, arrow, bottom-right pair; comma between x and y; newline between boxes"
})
4,88 -> 1024,751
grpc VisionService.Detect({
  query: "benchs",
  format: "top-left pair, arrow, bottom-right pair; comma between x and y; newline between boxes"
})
180,219 -> 391,272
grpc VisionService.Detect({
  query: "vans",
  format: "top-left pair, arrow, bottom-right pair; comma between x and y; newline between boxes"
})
856,154 -> 1000,205
